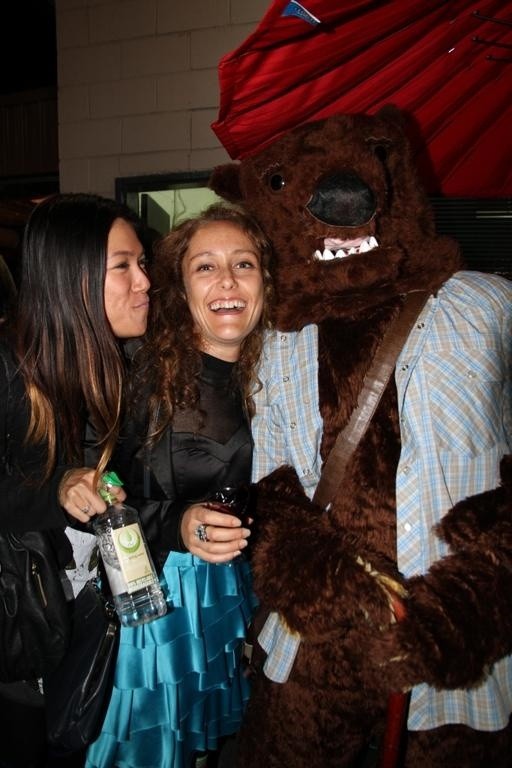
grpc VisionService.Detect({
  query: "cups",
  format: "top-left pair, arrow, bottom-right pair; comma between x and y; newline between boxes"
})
203,487 -> 252,567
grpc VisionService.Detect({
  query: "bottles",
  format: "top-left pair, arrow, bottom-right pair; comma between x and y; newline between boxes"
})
91,485 -> 167,630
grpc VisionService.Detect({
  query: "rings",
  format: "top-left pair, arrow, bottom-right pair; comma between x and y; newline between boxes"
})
82,505 -> 92,514
196,524 -> 210,543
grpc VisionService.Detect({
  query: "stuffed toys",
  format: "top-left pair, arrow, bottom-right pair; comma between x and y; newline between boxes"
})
206,99 -> 512,768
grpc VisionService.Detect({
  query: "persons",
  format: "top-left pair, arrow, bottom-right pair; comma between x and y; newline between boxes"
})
84,202 -> 279,768
1,191 -> 155,768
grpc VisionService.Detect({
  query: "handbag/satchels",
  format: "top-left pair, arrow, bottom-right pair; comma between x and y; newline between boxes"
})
0,530 -> 78,686
